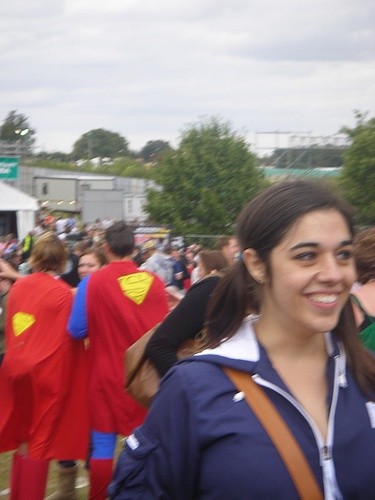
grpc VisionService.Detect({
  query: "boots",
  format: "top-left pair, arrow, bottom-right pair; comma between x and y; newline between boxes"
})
88,456 -> 115,500
9,451 -> 50,500
42,462 -> 80,500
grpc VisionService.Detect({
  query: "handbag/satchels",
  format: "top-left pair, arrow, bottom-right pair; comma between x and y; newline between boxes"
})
125,319 -> 205,407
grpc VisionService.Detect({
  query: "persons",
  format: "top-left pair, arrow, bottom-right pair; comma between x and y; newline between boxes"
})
107,178 -> 375,500
0,215 -> 375,500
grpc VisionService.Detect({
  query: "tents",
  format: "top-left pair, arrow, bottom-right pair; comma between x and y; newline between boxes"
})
0,183 -> 39,242
